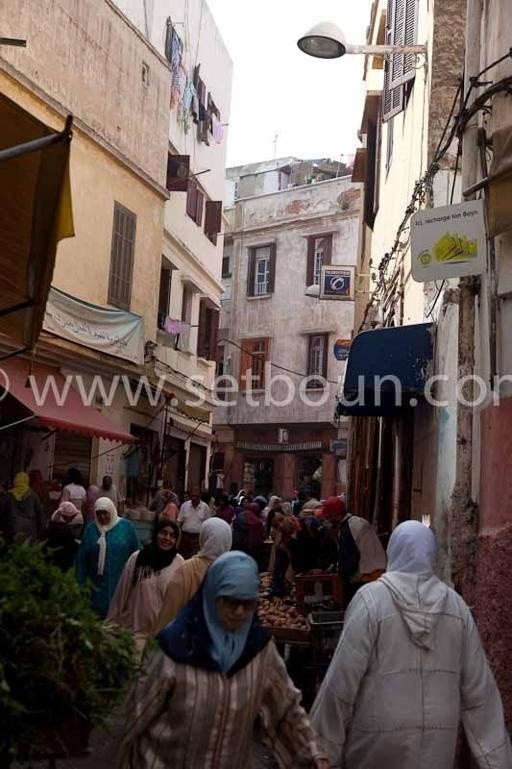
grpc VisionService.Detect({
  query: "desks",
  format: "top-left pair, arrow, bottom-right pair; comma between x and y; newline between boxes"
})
292,563 -> 344,608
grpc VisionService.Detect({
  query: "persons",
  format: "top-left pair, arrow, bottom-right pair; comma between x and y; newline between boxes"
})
88,549 -> 331,768
100,516 -> 187,682
306,519 -> 511,767
310,498 -> 388,605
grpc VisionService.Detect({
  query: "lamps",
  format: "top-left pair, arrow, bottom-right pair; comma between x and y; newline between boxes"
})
295,20 -> 427,67
301,282 -> 321,301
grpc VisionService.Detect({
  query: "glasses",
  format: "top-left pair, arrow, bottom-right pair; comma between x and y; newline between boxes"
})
220,598 -> 257,610
